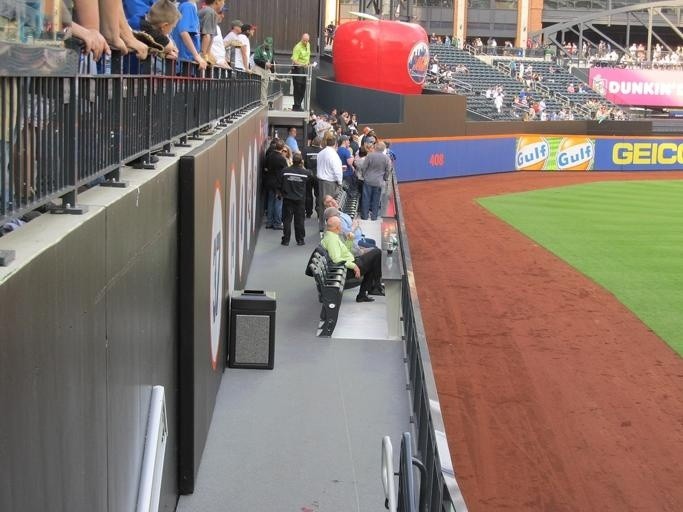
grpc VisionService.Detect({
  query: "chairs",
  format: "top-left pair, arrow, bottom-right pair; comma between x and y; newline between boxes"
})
308,179 -> 360,337
419,43 -> 631,122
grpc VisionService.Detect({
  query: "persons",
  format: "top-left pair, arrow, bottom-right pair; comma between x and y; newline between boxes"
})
319,216 -> 385,302
423,32 -> 683,124
326,19 -> 334,45
324,207 -> 386,290
290,33 -> 311,112
261,106 -> 396,250
1,1 -> 276,230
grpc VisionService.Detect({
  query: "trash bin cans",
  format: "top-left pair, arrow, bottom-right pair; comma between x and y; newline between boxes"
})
228,289 -> 276,370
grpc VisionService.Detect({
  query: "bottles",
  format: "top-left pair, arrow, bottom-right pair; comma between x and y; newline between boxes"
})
386,243 -> 392,255
392,236 -> 397,249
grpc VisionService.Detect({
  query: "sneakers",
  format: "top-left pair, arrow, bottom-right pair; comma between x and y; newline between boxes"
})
266,223 -> 273,229
273,224 -> 284,229
306,214 -> 311,218
297,240 -> 305,245
361,216 -> 377,220
292,104 -> 304,111
356,295 -> 374,302
281,240 -> 290,246
369,284 -> 384,296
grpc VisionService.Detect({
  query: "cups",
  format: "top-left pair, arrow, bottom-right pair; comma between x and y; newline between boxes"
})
389,233 -> 395,243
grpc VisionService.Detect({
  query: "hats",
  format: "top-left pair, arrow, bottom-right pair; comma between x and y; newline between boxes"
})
363,126 -> 374,137
339,135 -> 351,141
